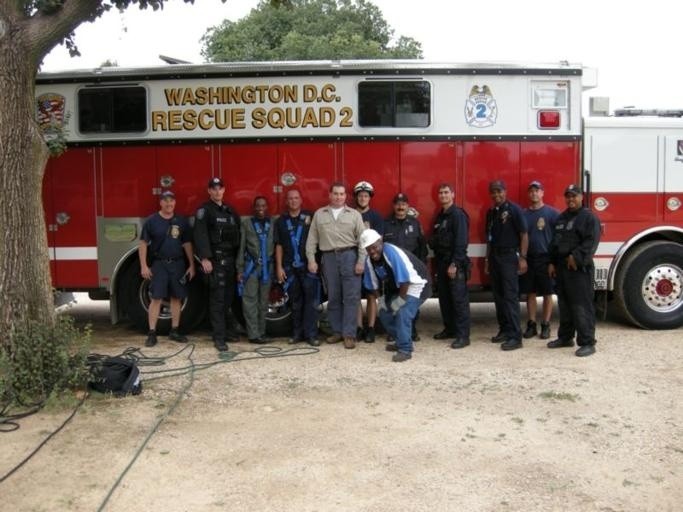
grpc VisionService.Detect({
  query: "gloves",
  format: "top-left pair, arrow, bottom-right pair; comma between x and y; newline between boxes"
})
390,296 -> 407,316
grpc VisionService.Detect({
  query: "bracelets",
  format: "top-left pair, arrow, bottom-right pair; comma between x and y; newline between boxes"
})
520,253 -> 527,262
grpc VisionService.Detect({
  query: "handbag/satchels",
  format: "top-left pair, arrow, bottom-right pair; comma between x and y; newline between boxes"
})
85,353 -> 140,395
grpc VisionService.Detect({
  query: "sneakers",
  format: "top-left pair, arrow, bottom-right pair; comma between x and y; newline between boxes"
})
356,326 -> 364,343
287,335 -> 303,344
364,327 -> 375,343
224,334 -> 241,342
547,338 -> 575,348
522,320 -> 537,339
386,335 -> 395,342
304,335 -> 320,346
491,335 -> 511,343
248,337 -> 265,344
145,329 -> 158,347
575,345 -> 596,357
539,320 -> 551,339
385,343 -> 398,352
451,338 -> 470,349
168,328 -> 188,343
412,336 -> 420,341
501,339 -> 522,351
215,341 -> 228,352
326,334 -> 342,344
261,334 -> 275,343
433,331 -> 458,340
343,335 -> 356,349
392,352 -> 412,362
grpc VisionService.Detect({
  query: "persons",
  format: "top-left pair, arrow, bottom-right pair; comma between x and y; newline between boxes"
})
547,185 -> 603,356
375,190 -> 429,342
354,179 -> 381,346
484,182 -> 531,352
359,228 -> 432,362
193,177 -> 243,351
274,185 -> 322,346
235,194 -> 278,344
137,191 -> 196,349
428,184 -> 474,350
520,184 -> 563,339
305,182 -> 367,348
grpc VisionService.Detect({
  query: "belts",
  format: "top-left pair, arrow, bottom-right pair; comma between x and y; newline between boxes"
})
321,246 -> 358,253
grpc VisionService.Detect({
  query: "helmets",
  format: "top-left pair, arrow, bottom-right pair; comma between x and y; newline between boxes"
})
353,180 -> 375,198
360,229 -> 381,250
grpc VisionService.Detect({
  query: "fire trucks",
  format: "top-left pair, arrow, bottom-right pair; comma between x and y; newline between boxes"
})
30,55 -> 682,333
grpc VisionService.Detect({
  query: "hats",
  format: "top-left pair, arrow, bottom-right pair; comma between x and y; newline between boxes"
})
489,179 -> 504,192
527,180 -> 544,191
392,193 -> 408,205
160,189 -> 176,200
564,184 -> 581,196
207,177 -> 225,187
